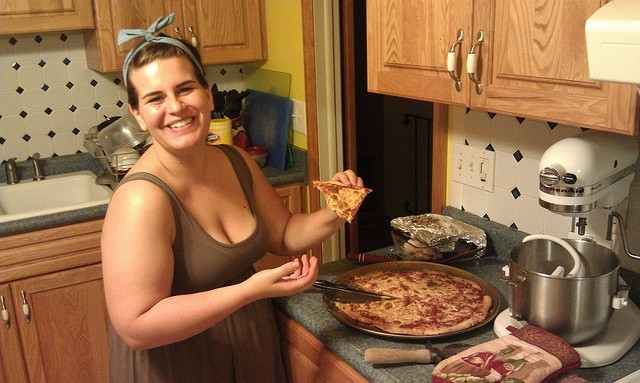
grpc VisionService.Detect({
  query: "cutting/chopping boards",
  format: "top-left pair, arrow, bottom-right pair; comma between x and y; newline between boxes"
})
243,89 -> 292,172
243,66 -> 291,98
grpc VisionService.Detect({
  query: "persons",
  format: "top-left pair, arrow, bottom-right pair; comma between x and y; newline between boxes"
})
100,13 -> 364,382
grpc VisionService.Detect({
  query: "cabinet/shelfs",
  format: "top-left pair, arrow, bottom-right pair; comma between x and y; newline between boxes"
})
253,179 -> 304,271
275,307 -> 369,382
365,1 -> 640,137
83,0 -> 270,73
0,1 -> 94,37
1,218 -> 112,382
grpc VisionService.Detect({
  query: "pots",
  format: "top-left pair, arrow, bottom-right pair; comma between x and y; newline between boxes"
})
93,113 -> 148,159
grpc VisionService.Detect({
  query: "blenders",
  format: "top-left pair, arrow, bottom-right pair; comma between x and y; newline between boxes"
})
492,129 -> 640,368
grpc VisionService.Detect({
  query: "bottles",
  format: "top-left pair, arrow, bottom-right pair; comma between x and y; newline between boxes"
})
206,118 -> 233,146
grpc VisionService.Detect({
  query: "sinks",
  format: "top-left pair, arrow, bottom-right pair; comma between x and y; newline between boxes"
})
0,170 -> 114,223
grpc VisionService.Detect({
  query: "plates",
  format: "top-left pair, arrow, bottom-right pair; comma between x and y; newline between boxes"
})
328,258 -> 501,341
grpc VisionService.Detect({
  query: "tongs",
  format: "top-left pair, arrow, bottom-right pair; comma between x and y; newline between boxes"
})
311,279 -> 392,300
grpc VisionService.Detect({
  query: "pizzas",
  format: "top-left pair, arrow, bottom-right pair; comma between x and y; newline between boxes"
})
313,179 -> 374,225
330,269 -> 493,336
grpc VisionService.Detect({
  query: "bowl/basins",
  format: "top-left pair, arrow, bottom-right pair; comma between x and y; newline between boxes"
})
245,145 -> 268,168
111,147 -> 139,171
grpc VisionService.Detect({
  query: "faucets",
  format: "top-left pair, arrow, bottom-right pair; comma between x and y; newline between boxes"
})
5,159 -> 20,185
